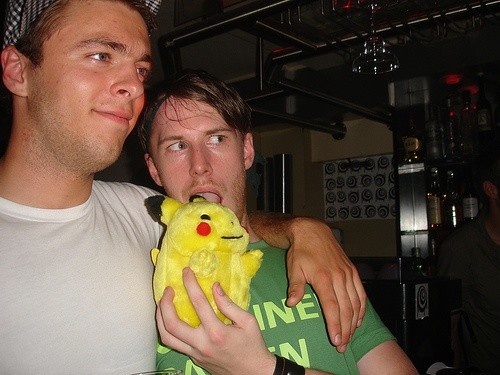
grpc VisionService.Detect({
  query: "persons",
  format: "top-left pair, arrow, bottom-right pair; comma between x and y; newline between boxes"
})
1,1 -> 366,375
438,148 -> 500,375
133,72 -> 421,375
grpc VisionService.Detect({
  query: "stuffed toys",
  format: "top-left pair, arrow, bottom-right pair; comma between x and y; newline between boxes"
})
146,195 -> 264,328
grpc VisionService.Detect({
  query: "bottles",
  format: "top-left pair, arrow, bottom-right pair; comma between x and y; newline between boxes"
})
424,71 -> 492,162
427,166 -> 480,232
407,246 -> 429,323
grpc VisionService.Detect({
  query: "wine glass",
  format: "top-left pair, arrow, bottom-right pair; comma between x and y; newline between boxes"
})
351,0 -> 400,75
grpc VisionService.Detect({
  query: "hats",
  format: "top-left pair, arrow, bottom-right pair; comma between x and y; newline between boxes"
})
0,0 -> 161,51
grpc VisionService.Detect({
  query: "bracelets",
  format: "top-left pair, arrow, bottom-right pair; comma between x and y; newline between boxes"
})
273,353 -> 305,375
